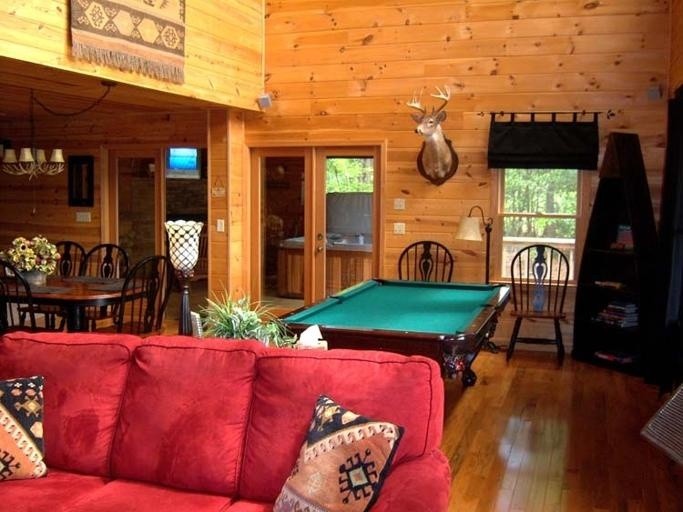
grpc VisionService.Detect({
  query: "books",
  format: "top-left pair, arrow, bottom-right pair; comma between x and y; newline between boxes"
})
593,224 -> 637,329
594,349 -> 639,364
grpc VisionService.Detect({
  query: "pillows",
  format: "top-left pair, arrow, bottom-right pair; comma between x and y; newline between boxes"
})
0,374 -> 47,483
271,391 -> 404,510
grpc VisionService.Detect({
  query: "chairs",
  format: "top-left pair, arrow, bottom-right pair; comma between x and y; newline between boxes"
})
56,243 -> 129,331
397,238 -> 454,282
15,240 -> 86,333
0,260 -> 35,333
505,241 -> 569,369
92,254 -> 174,335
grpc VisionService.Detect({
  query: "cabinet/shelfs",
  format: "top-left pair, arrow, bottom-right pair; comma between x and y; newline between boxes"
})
570,132 -> 660,377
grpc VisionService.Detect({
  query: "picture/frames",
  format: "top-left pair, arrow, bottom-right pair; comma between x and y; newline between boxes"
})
66,155 -> 96,208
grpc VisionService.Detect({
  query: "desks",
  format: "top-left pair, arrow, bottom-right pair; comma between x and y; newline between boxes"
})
0,274 -> 162,332
274,277 -> 509,391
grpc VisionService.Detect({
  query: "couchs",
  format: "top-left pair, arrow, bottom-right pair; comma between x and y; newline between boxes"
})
0,330 -> 454,511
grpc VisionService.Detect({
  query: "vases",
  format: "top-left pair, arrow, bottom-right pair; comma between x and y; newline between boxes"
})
13,271 -> 48,291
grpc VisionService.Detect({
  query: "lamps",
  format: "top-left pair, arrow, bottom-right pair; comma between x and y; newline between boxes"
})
0,144 -> 65,181
452,203 -> 495,283
166,219 -> 204,336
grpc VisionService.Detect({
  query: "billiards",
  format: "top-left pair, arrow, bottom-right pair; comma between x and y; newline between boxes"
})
442,352 -> 468,379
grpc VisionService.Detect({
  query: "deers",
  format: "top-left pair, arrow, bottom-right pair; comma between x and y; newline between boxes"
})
405,83 -> 456,184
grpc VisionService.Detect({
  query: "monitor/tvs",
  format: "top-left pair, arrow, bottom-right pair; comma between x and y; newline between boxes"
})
165,147 -> 200,180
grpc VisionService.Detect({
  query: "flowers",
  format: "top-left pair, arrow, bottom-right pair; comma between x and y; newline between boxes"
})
0,234 -> 61,278
